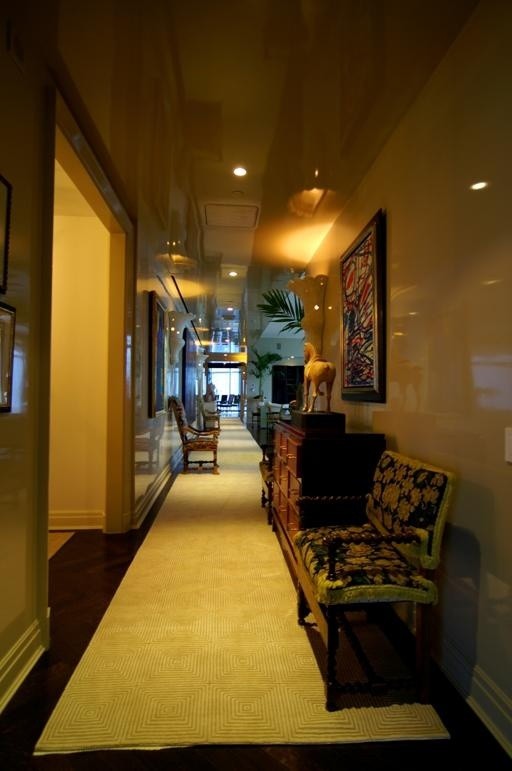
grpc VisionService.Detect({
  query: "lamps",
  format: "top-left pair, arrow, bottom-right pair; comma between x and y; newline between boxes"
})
197,354 -> 209,380
287,274 -> 327,353
168,311 -> 196,364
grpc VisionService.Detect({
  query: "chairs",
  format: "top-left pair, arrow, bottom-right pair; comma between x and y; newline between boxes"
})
134,416 -> 165,473
215,395 -> 240,412
171,395 -> 220,474
197,395 -> 220,431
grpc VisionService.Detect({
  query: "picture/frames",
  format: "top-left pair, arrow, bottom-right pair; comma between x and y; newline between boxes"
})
338,207 -> 386,404
0,174 -> 12,296
148,290 -> 167,418
0,301 -> 17,413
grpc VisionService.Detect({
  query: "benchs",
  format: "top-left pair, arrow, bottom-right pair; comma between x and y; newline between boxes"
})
259,443 -> 274,526
293,449 -> 457,711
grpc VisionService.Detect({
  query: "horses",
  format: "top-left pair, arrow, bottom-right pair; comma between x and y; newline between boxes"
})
302,342 -> 337,413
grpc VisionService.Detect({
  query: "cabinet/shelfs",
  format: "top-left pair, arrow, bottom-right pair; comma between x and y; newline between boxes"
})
270,419 -> 386,595
272,365 -> 304,404
203,400 -> 218,429
